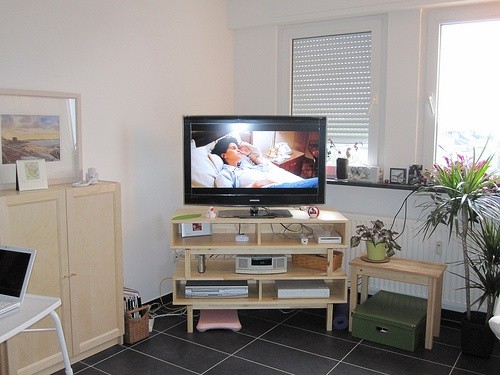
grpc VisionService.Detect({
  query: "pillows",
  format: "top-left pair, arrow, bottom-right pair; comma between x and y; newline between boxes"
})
192,131 -> 243,188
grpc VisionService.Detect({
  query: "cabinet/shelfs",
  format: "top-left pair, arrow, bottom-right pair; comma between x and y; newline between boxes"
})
0,176 -> 124,375
168,207 -> 350,335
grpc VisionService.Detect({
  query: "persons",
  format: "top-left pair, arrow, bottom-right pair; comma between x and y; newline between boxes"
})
210,137 -> 319,188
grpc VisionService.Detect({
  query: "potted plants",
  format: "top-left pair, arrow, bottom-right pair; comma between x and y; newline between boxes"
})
392,125 -> 500,360
327,134 -> 368,180
351,218 -> 402,261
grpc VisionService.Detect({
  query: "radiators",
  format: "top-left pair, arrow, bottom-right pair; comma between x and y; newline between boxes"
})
284,211 -> 500,318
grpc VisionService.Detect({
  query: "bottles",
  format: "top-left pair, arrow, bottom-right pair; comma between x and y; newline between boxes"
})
198,255 -> 205,273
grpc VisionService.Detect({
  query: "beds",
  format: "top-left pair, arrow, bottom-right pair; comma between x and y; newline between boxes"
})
191,131 -> 320,189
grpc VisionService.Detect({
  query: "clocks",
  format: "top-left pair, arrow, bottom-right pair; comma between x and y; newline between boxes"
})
389,167 -> 408,185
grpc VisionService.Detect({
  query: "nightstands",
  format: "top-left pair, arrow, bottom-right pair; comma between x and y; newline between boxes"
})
261,144 -> 306,177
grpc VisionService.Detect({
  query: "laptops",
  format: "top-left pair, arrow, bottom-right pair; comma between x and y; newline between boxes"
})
0,245 -> 38,315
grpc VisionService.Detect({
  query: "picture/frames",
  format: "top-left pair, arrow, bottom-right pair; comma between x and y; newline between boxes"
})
0,86 -> 83,190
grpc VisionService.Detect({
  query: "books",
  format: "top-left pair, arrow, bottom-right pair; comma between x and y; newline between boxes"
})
123,287 -> 143,319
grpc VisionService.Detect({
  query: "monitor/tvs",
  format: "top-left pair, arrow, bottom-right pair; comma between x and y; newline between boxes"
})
182,115 -> 327,219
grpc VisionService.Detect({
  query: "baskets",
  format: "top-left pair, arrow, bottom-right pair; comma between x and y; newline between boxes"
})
291,251 -> 343,271
124,305 -> 150,344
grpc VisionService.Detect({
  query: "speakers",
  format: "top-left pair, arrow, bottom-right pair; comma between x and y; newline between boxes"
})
337,158 -> 349,179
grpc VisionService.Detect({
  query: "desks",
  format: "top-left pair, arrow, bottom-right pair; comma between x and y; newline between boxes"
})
346,253 -> 447,350
0,292 -> 76,375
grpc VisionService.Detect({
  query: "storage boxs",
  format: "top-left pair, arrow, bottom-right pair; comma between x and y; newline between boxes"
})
349,290 -> 428,353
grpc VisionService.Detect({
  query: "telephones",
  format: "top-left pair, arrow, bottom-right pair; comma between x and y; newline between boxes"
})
86,167 -> 99,185
